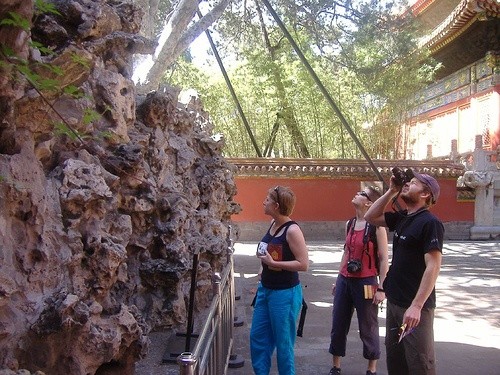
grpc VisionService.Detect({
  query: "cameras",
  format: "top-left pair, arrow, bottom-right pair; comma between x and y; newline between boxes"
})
392,166 -> 414,186
258,242 -> 268,254
347,259 -> 361,273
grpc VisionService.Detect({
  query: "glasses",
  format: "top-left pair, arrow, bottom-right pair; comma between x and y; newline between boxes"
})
275,184 -> 282,205
361,189 -> 373,203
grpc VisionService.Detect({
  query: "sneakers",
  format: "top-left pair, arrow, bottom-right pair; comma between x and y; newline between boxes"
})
328,366 -> 377,375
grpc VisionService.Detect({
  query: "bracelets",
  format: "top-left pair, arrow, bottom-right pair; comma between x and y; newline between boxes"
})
376,288 -> 385,292
332,283 -> 337,286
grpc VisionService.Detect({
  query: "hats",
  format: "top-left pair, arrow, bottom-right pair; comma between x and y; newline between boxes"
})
414,171 -> 440,203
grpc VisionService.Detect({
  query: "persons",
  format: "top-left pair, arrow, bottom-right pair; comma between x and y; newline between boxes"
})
250,186 -> 309,375
328,184 -> 389,375
364,171 -> 445,375
